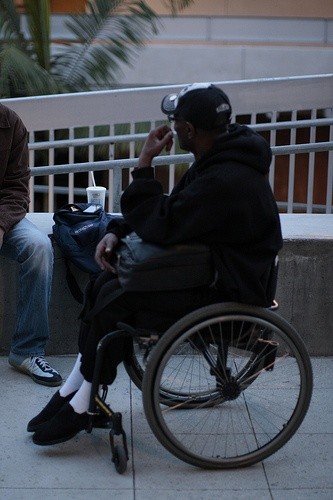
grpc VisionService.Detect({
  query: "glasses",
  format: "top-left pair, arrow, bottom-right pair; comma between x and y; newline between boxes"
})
167,114 -> 190,125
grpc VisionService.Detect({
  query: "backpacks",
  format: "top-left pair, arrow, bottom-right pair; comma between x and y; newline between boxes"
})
53,203 -> 124,303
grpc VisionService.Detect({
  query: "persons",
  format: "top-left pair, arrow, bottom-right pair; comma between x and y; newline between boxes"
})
0,103 -> 64,386
25,84 -> 279,445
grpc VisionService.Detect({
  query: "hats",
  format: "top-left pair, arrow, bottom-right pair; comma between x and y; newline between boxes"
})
161,81 -> 234,130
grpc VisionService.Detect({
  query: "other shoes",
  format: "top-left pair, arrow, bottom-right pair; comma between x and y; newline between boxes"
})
25,389 -> 80,434
29,403 -> 101,448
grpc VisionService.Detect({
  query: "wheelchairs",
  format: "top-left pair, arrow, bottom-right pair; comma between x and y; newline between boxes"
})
90,257 -> 314,475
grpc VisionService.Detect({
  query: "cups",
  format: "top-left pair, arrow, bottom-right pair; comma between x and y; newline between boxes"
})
87,187 -> 105,209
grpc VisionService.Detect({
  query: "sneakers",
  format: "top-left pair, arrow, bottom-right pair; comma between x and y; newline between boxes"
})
9,354 -> 63,385
